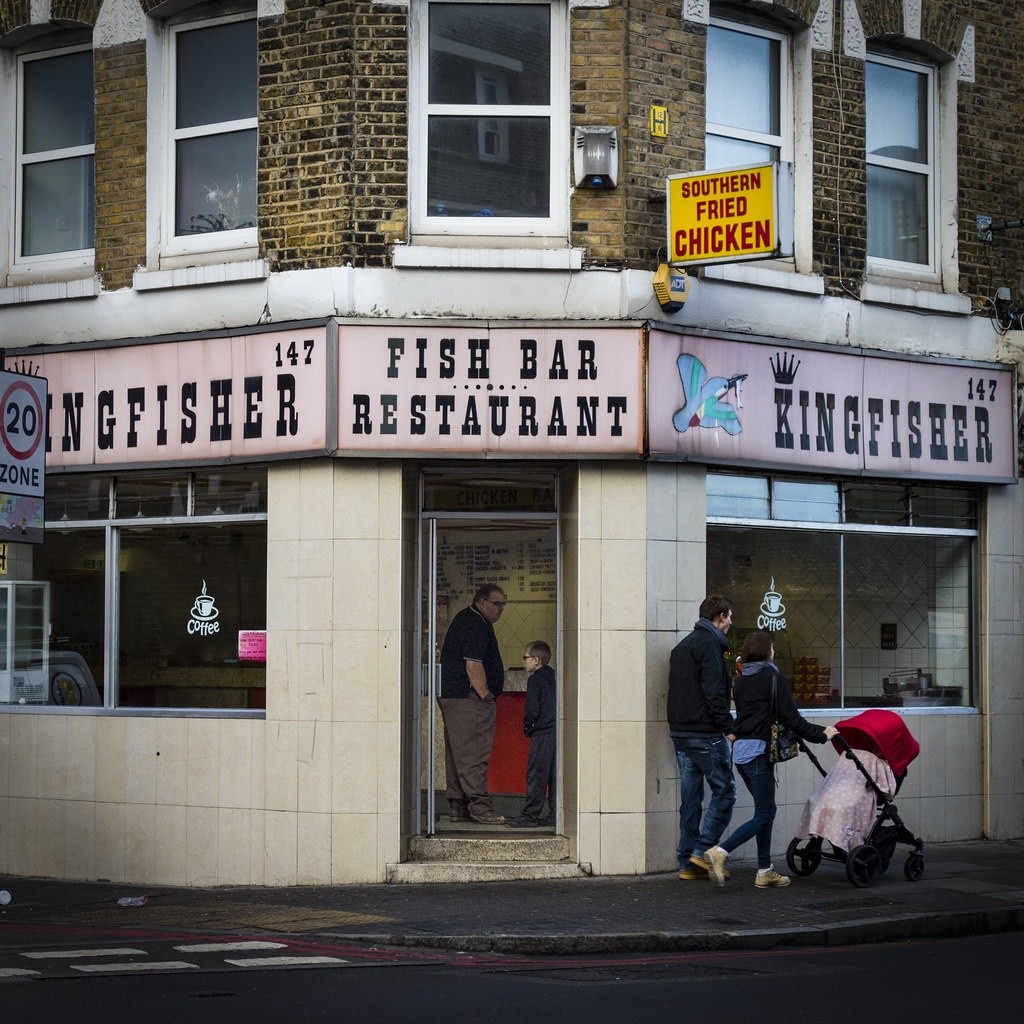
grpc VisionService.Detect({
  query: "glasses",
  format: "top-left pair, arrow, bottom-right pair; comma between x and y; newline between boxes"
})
523,656 -> 534,660
484,598 -> 506,606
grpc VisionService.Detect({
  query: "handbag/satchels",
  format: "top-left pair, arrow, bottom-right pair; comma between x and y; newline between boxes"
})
769,720 -> 798,763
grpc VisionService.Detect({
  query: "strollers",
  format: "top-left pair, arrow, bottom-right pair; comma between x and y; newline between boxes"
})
785,711 -> 928,883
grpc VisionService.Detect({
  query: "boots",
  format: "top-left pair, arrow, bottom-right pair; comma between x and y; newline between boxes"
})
703,845 -> 729,888
754,863 -> 791,890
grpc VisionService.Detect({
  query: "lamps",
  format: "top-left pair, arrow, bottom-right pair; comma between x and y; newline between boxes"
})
207,486 -> 229,529
53,488 -> 77,535
128,480 -> 153,532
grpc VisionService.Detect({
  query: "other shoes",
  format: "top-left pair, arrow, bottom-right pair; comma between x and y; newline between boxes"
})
508,817 -> 536,827
688,849 -> 730,879
680,867 -> 709,880
539,818 -> 555,826
470,807 -> 506,824
450,810 -> 474,822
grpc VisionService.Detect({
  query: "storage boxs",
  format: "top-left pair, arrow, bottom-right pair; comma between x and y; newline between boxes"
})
791,658 -> 830,704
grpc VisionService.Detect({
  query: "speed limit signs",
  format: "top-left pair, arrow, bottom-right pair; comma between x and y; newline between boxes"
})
1,370 -> 48,545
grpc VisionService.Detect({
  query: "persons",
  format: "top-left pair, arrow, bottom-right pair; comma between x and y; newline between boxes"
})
507,640 -> 557,828
703,632 -> 840,887
435,581 -> 506,824
665,594 -> 735,880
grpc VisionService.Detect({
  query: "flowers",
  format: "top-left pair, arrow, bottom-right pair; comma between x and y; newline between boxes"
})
199,174 -> 258,230
